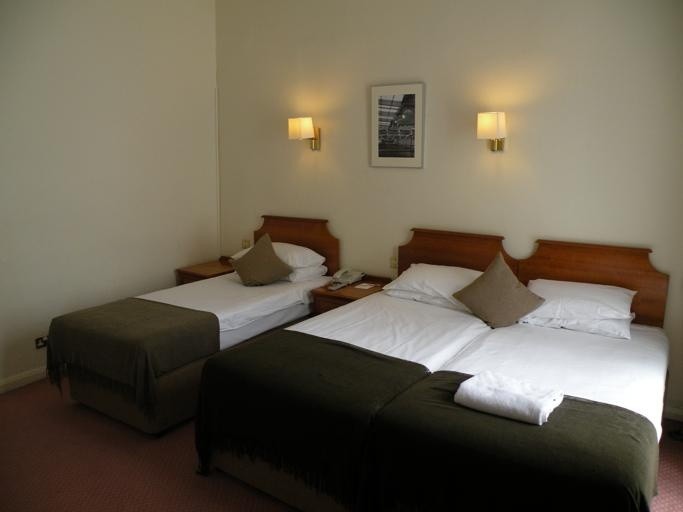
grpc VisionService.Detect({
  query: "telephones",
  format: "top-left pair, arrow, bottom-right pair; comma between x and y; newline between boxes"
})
331,268 -> 365,285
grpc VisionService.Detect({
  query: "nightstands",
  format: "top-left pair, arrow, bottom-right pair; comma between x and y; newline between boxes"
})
174,256 -> 235,286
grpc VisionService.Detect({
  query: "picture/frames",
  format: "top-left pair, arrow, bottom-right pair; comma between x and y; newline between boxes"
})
370,82 -> 422,169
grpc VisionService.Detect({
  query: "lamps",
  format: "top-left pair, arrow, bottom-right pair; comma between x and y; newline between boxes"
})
476,111 -> 506,153
286,116 -> 321,153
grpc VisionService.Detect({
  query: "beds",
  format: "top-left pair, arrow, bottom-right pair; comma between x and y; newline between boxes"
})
48,213 -> 340,442
